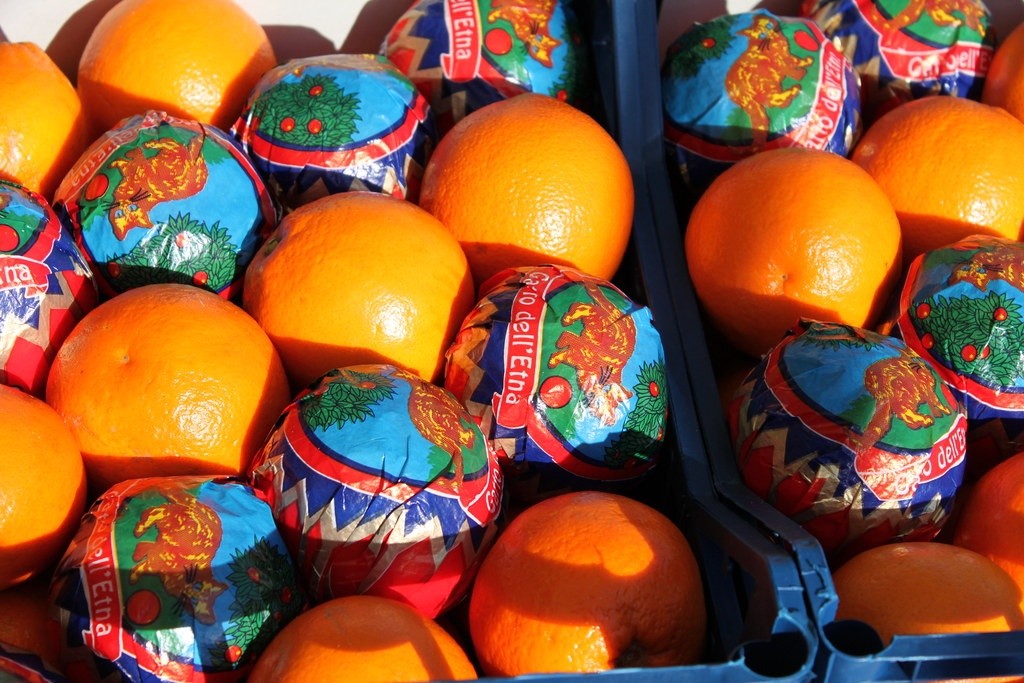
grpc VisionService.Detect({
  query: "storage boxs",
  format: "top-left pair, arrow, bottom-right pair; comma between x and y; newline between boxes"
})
637,0 -> 1023,683
432,1 -> 819,683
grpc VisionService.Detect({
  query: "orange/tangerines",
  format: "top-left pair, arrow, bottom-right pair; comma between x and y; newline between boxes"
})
0,0 -> 1024,683
